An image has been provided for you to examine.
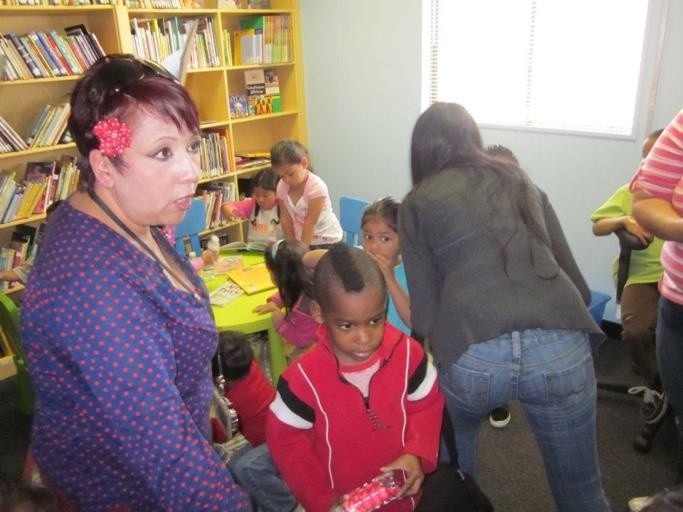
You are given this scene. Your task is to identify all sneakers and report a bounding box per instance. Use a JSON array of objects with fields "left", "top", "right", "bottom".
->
[
  {"left": 488, "top": 406, "right": 512, "bottom": 429},
  {"left": 627, "top": 385, "right": 669, "bottom": 425}
]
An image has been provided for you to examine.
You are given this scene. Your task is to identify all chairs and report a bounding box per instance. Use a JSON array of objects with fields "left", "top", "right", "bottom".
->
[
  {"left": 0, "top": 293, "right": 35, "bottom": 417},
  {"left": 173, "top": 199, "right": 206, "bottom": 259},
  {"left": 596, "top": 226, "right": 668, "bottom": 451},
  {"left": 338, "top": 197, "right": 369, "bottom": 246}
]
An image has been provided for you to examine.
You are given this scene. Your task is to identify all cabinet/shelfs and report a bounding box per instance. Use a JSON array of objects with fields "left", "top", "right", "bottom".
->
[
  {"left": 126, "top": 1, "right": 307, "bottom": 242},
  {"left": 0, "top": 3, "right": 125, "bottom": 384}
]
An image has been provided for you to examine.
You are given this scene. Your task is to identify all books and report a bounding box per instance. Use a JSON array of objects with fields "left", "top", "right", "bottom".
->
[
  {"left": 199, "top": 130, "right": 230, "bottom": 180},
  {"left": 195, "top": 180, "right": 236, "bottom": 229},
  {"left": 129, "top": 17, "right": 222, "bottom": 68},
  {"left": 0, "top": 101, "right": 71, "bottom": 154},
  {"left": 226, "top": 263, "right": 278, "bottom": 295},
  {"left": 220, "top": 240, "right": 269, "bottom": 252},
  {"left": 222, "top": 13, "right": 292, "bottom": 66},
  {"left": 0, "top": 22, "right": 106, "bottom": 81},
  {"left": 1, "top": 225, "right": 39, "bottom": 288},
  {"left": 0, "top": 155, "right": 81, "bottom": 224}
]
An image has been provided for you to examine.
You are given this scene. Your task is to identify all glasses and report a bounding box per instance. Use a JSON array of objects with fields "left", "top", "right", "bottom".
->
[{"left": 86, "top": 56, "right": 181, "bottom": 148}]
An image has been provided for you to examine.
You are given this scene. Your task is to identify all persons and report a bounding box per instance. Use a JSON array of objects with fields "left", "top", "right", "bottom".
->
[
  {"left": 220, "top": 167, "right": 284, "bottom": 241},
  {"left": 253, "top": 238, "right": 319, "bottom": 347},
  {"left": 270, "top": 139, "right": 344, "bottom": 250},
  {"left": 590, "top": 129, "right": 665, "bottom": 390},
  {"left": 265, "top": 242, "right": 444, "bottom": 512},
  {"left": 396, "top": 101, "right": 614, "bottom": 512},
  {"left": 21, "top": 54, "right": 251, "bottom": 512},
  {"left": 0, "top": 199, "right": 64, "bottom": 286},
  {"left": 211, "top": 331, "right": 297, "bottom": 512},
  {"left": 353, "top": 198, "right": 413, "bottom": 339},
  {"left": 483, "top": 145, "right": 590, "bottom": 430},
  {"left": 628, "top": 109, "right": 683, "bottom": 512},
  {"left": 191, "top": 248, "right": 218, "bottom": 273}
]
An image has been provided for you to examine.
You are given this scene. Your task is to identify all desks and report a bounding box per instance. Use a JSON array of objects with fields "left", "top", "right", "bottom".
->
[{"left": 183, "top": 249, "right": 289, "bottom": 389}]
